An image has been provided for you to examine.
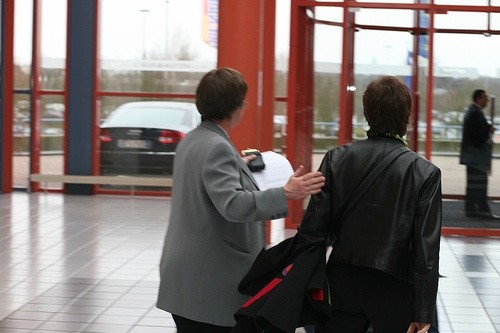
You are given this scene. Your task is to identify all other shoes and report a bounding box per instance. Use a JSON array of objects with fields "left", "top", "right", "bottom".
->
[{"left": 465, "top": 211, "right": 500, "bottom": 220}]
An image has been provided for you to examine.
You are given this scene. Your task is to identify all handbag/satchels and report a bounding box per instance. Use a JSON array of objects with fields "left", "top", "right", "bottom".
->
[{"left": 237, "top": 235, "right": 303, "bottom": 295}]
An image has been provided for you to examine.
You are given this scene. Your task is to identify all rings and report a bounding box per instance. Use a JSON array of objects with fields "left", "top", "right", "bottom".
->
[{"left": 307, "top": 187, "right": 309, "bottom": 191}]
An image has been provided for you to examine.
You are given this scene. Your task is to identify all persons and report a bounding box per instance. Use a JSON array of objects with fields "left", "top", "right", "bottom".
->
[
  {"left": 459, "top": 89, "right": 500, "bottom": 220},
  {"left": 297, "top": 75, "right": 442, "bottom": 333},
  {"left": 155, "top": 68, "right": 326, "bottom": 333}
]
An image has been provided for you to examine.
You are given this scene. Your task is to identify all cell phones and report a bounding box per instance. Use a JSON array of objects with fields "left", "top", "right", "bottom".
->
[{"left": 245, "top": 151, "right": 265, "bottom": 171}]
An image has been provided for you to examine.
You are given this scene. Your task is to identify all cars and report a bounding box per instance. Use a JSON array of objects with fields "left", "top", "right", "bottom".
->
[{"left": 100, "top": 101, "right": 202, "bottom": 178}]
